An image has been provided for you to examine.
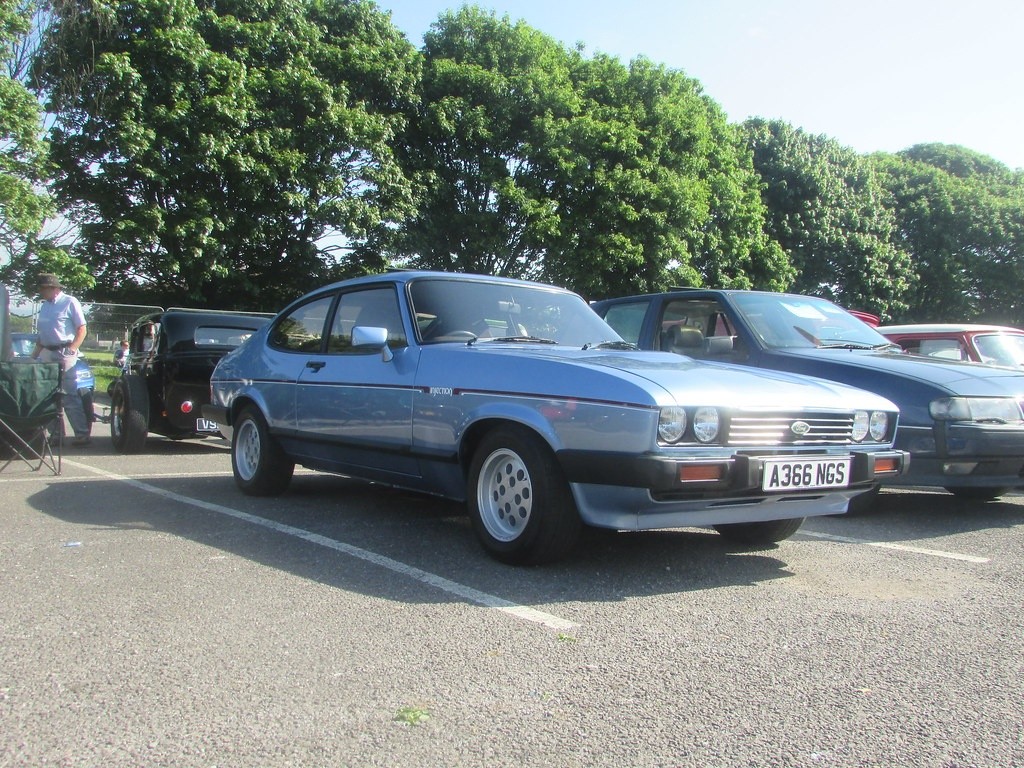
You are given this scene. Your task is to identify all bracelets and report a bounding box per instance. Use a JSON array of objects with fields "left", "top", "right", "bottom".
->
[{"left": 66, "top": 346, "right": 75, "bottom": 355}]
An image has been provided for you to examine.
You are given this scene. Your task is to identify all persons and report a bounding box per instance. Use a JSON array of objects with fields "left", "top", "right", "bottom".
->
[
  {"left": 113, "top": 340, "right": 129, "bottom": 367},
  {"left": 28, "top": 274, "right": 91, "bottom": 446},
  {"left": 143, "top": 310, "right": 155, "bottom": 352}
]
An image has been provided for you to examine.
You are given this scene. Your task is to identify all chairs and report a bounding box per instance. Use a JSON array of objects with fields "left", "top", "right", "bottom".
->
[
  {"left": 354, "top": 298, "right": 408, "bottom": 349},
  {"left": 197, "top": 338, "right": 212, "bottom": 344},
  {"left": 661, "top": 325, "right": 705, "bottom": 361},
  {"left": 1, "top": 359, "right": 65, "bottom": 475},
  {"left": 422, "top": 303, "right": 493, "bottom": 344},
  {"left": 226, "top": 336, "right": 243, "bottom": 345}
]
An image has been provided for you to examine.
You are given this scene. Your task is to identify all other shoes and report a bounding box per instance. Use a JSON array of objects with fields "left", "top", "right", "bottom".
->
[
  {"left": 45, "top": 437, "right": 65, "bottom": 446},
  {"left": 72, "top": 437, "right": 91, "bottom": 446}
]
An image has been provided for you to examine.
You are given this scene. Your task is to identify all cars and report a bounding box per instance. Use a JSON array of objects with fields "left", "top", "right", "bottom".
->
[
  {"left": 588, "top": 290, "right": 1024, "bottom": 520},
  {"left": 196, "top": 269, "right": 914, "bottom": 570},
  {"left": 874, "top": 323, "right": 1024, "bottom": 370},
  {"left": 105, "top": 309, "right": 274, "bottom": 455},
  {"left": 663, "top": 305, "right": 881, "bottom": 337},
  {"left": 12, "top": 332, "right": 96, "bottom": 396}
]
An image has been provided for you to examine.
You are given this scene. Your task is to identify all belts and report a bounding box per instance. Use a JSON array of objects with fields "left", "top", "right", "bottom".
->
[{"left": 42, "top": 342, "right": 72, "bottom": 351}]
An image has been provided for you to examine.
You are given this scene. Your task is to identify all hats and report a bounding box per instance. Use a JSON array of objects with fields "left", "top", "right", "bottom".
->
[{"left": 34, "top": 274, "right": 66, "bottom": 289}]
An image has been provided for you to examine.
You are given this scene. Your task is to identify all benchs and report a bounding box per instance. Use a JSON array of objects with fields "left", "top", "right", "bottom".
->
[{"left": 704, "top": 335, "right": 749, "bottom": 363}]
